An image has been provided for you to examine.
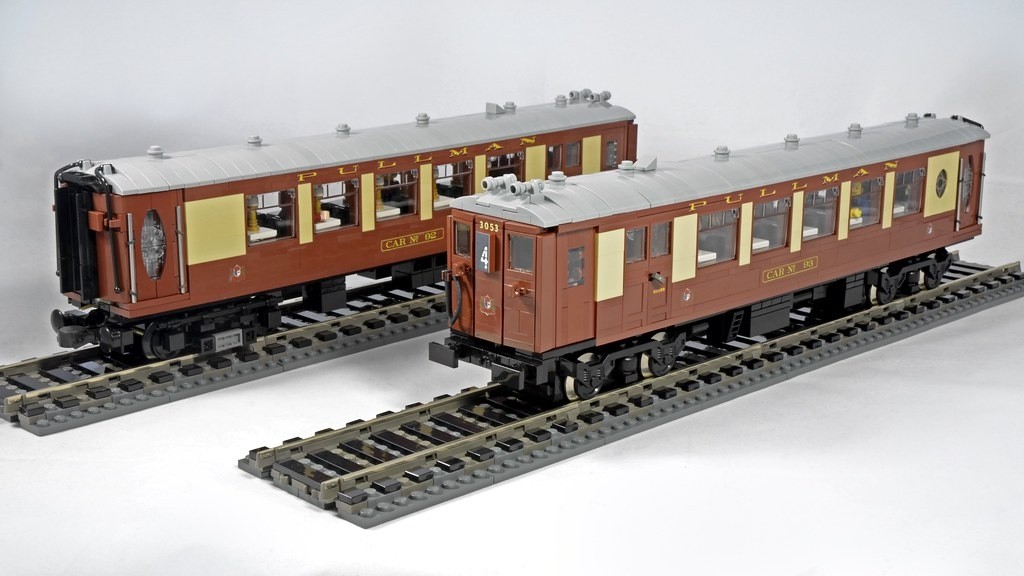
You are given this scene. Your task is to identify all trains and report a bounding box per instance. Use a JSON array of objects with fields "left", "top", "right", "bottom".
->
[
  {"left": 49, "top": 88, "right": 640, "bottom": 364},
  {"left": 428, "top": 112, "right": 991, "bottom": 405}
]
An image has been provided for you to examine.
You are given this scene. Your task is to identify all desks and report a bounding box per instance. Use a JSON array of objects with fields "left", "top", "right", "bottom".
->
[
  {"left": 313, "top": 216, "right": 342, "bottom": 231},
  {"left": 699, "top": 248, "right": 718, "bottom": 262},
  {"left": 247, "top": 225, "right": 277, "bottom": 241},
  {"left": 434, "top": 195, "right": 455, "bottom": 209},
  {"left": 375, "top": 205, "right": 401, "bottom": 218},
  {"left": 751, "top": 237, "right": 771, "bottom": 249},
  {"left": 892, "top": 204, "right": 906, "bottom": 216},
  {"left": 802, "top": 225, "right": 818, "bottom": 238},
  {"left": 851, "top": 216, "right": 863, "bottom": 226}
]
